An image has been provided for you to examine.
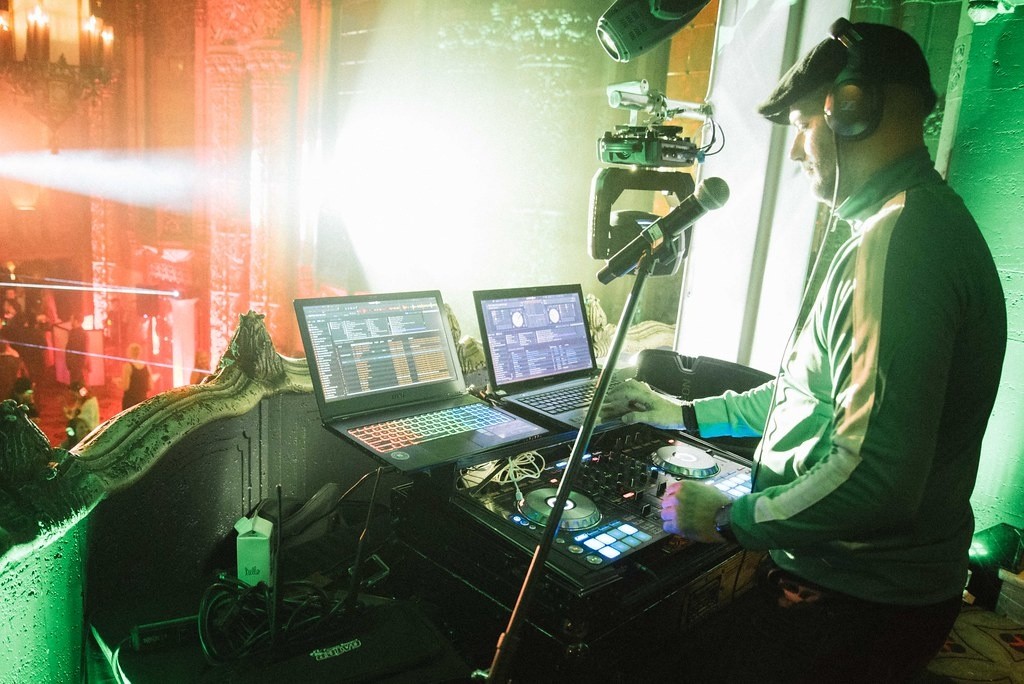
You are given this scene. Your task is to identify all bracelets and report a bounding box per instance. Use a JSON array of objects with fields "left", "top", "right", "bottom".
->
[{"left": 681, "top": 403, "right": 699, "bottom": 433}]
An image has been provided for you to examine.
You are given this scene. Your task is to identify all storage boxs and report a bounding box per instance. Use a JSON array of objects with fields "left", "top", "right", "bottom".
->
[{"left": 238, "top": 508, "right": 276, "bottom": 587}]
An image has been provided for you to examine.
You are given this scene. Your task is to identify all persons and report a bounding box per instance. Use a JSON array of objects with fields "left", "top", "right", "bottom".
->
[
  {"left": 0, "top": 286, "right": 50, "bottom": 408},
  {"left": 599, "top": 17, "right": 1009, "bottom": 684},
  {"left": 111, "top": 341, "right": 153, "bottom": 412},
  {"left": 64, "top": 379, "right": 100, "bottom": 430},
  {"left": 6, "top": 376, "right": 41, "bottom": 424},
  {"left": 64, "top": 310, "right": 94, "bottom": 396},
  {"left": 191, "top": 347, "right": 214, "bottom": 385},
  {"left": 58, "top": 418, "right": 91, "bottom": 451}
]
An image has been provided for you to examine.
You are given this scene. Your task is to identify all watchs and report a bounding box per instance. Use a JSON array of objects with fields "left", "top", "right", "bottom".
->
[{"left": 712, "top": 502, "right": 739, "bottom": 545}]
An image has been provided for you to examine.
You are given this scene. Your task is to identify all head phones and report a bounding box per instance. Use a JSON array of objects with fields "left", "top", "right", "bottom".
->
[{"left": 823, "top": 17, "right": 885, "bottom": 141}]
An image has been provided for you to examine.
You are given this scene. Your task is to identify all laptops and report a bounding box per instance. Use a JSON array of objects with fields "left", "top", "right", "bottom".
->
[
  {"left": 471, "top": 284, "right": 649, "bottom": 431},
  {"left": 293, "top": 290, "right": 549, "bottom": 477}
]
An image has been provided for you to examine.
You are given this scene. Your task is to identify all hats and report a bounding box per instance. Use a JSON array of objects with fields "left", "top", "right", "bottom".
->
[{"left": 759, "top": 22, "right": 932, "bottom": 125}]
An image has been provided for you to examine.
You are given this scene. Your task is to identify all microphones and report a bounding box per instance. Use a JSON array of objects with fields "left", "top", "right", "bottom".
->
[{"left": 596, "top": 177, "right": 730, "bottom": 285}]
